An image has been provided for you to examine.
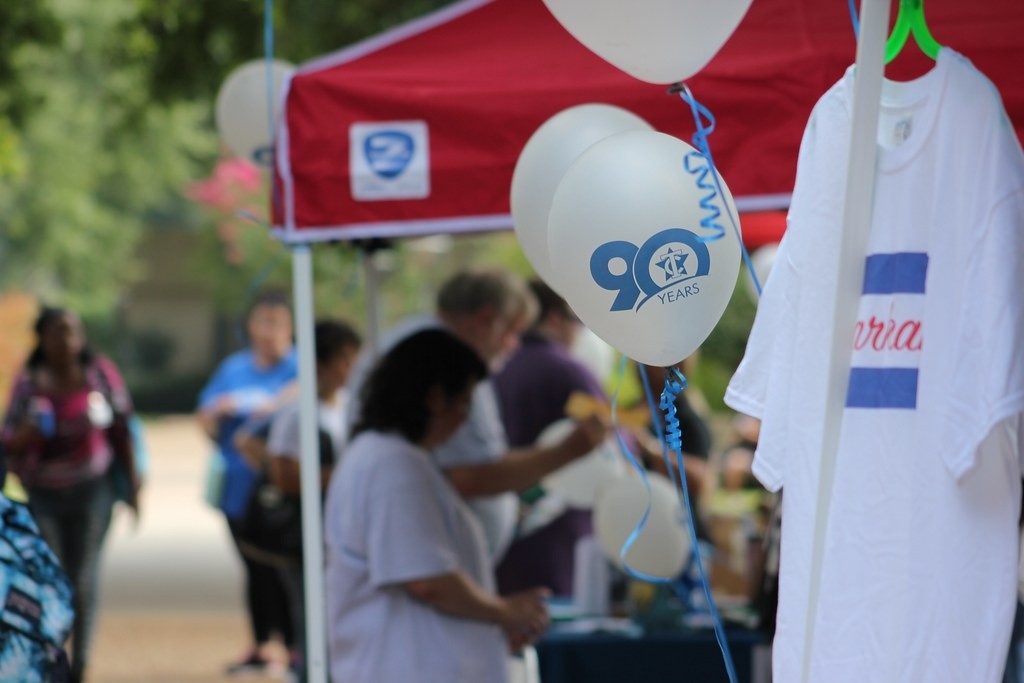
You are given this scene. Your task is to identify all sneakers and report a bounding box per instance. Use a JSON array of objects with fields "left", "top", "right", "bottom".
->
[{"left": 228, "top": 654, "right": 269, "bottom": 678}]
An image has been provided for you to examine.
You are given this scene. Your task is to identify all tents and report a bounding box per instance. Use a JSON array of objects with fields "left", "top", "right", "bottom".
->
[{"left": 269, "top": 1, "right": 1024, "bottom": 244}]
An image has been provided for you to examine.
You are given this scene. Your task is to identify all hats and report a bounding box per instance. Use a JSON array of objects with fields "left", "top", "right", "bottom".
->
[{"left": 530, "top": 279, "right": 584, "bottom": 321}]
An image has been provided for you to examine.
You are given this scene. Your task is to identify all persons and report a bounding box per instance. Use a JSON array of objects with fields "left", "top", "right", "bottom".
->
[
  {"left": 0, "top": 306, "right": 149, "bottom": 681},
  {"left": 194, "top": 270, "right": 778, "bottom": 683},
  {"left": 0, "top": 468, "right": 81, "bottom": 683}
]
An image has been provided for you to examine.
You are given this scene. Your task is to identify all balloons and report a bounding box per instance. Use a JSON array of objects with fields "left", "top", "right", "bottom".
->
[
  {"left": 509, "top": 102, "right": 661, "bottom": 299},
  {"left": 551, "top": 131, "right": 744, "bottom": 367},
  {"left": 543, "top": 0, "right": 751, "bottom": 85},
  {"left": 214, "top": 55, "right": 297, "bottom": 167}
]
